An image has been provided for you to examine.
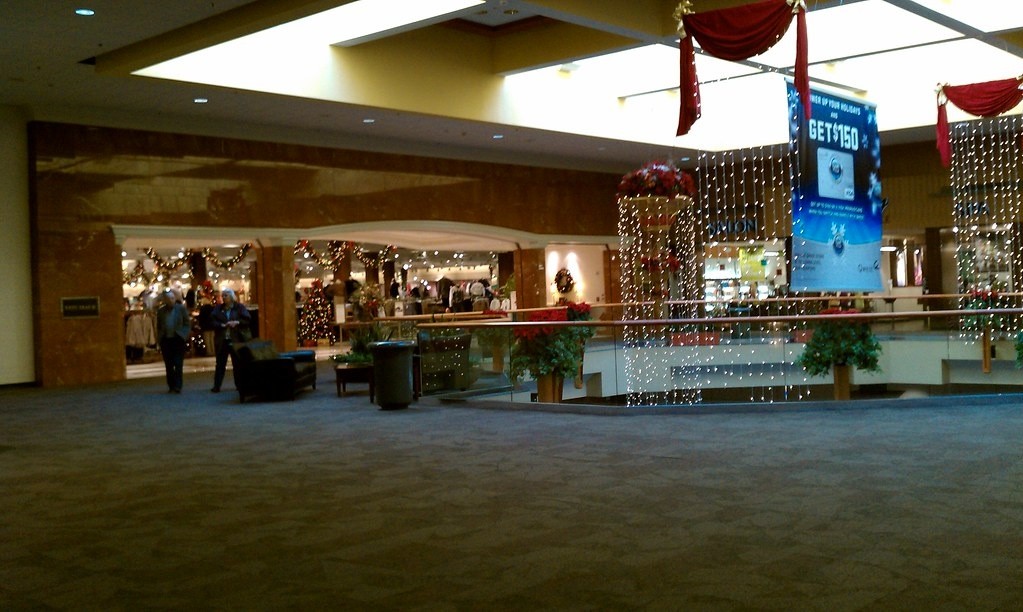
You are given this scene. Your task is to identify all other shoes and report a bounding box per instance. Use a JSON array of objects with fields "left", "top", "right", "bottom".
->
[
  {"left": 168, "top": 387, "right": 182, "bottom": 394},
  {"left": 211, "top": 384, "right": 220, "bottom": 391}
]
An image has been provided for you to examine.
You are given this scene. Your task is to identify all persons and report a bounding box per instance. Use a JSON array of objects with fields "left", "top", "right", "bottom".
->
[
  {"left": 959, "top": 279, "right": 969, "bottom": 307},
  {"left": 211, "top": 288, "right": 250, "bottom": 393},
  {"left": 389, "top": 278, "right": 400, "bottom": 297},
  {"left": 990, "top": 274, "right": 998, "bottom": 292},
  {"left": 156, "top": 292, "right": 189, "bottom": 393}
]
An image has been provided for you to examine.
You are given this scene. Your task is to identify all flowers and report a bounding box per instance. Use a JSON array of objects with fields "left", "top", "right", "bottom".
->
[
  {"left": 615, "top": 160, "right": 696, "bottom": 200},
  {"left": 554, "top": 268, "right": 576, "bottom": 294},
  {"left": 348, "top": 281, "right": 393, "bottom": 317}
]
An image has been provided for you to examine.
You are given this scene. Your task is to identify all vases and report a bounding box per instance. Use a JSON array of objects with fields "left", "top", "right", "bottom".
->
[
  {"left": 621, "top": 196, "right": 692, "bottom": 232},
  {"left": 353, "top": 303, "right": 371, "bottom": 322}
]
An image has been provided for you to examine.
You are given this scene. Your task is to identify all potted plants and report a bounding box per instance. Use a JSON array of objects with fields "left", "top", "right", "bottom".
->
[
  {"left": 664, "top": 325, "right": 720, "bottom": 345},
  {"left": 478, "top": 328, "right": 507, "bottom": 373},
  {"left": 789, "top": 321, "right": 815, "bottom": 342},
  {"left": 507, "top": 316, "right": 598, "bottom": 402}
]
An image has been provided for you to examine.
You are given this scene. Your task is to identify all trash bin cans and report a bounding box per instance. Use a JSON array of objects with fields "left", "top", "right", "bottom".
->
[
  {"left": 728, "top": 308, "right": 753, "bottom": 339},
  {"left": 366, "top": 340, "right": 418, "bottom": 410}
]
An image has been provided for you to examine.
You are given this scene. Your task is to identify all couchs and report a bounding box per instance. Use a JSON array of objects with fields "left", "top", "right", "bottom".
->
[
  {"left": 409, "top": 332, "right": 479, "bottom": 400},
  {"left": 228, "top": 339, "right": 317, "bottom": 405}
]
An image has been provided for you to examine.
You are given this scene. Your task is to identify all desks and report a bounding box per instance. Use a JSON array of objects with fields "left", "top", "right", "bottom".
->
[
  {"left": 340, "top": 321, "right": 401, "bottom": 353},
  {"left": 917, "top": 292, "right": 1023, "bottom": 328}
]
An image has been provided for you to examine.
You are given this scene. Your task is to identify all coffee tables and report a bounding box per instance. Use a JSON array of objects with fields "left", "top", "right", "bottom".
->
[{"left": 335, "top": 362, "right": 372, "bottom": 398}]
